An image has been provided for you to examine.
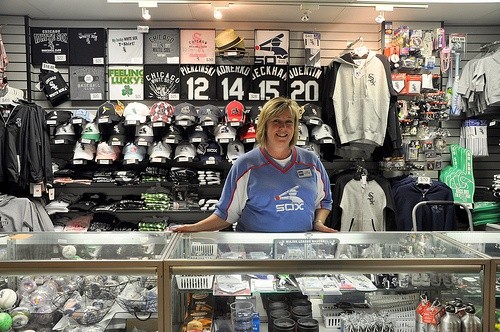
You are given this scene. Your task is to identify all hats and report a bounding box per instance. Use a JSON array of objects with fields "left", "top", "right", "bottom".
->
[
  {"left": 297, "top": 123, "right": 308, "bottom": 140},
  {"left": 298, "top": 104, "right": 323, "bottom": 122},
  {"left": 47, "top": 101, "right": 264, "bottom": 165},
  {"left": 311, "top": 124, "right": 334, "bottom": 141}
]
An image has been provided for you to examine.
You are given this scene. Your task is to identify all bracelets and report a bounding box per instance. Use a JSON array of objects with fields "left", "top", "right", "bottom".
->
[{"left": 314, "top": 220, "right": 321, "bottom": 224}]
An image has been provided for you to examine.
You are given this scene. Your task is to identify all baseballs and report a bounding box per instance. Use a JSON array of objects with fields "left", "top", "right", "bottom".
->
[
  {"left": 0, "top": 288, "right": 17, "bottom": 310},
  {"left": 0, "top": 312, "right": 12, "bottom": 332},
  {"left": 61, "top": 245, "right": 77, "bottom": 259},
  {"left": 9, "top": 307, "right": 31, "bottom": 328}
]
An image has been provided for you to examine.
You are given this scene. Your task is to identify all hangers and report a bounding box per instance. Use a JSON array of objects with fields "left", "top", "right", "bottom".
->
[
  {"left": 408, "top": 165, "right": 433, "bottom": 186},
  {"left": 350, "top": 37, "right": 370, "bottom": 58},
  {"left": 0, "top": 85, "right": 24, "bottom": 107}
]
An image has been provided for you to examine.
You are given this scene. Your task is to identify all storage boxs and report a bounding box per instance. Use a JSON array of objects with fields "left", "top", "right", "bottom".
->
[{"left": 323, "top": 289, "right": 366, "bottom": 305}]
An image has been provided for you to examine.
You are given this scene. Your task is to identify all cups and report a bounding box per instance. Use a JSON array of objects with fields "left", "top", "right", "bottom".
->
[
  {"left": 371, "top": 272, "right": 454, "bottom": 289},
  {"left": 230, "top": 301, "right": 254, "bottom": 332}
]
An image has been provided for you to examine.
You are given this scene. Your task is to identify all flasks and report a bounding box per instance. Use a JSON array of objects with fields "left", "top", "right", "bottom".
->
[{"left": 415, "top": 295, "right": 481, "bottom": 332}]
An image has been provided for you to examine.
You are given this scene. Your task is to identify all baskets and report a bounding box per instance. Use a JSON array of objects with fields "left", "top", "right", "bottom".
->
[
  {"left": 190, "top": 244, "right": 218, "bottom": 260},
  {"left": 319, "top": 303, "right": 372, "bottom": 320},
  {"left": 323, "top": 316, "right": 350, "bottom": 328},
  {"left": 367, "top": 293, "right": 420, "bottom": 332},
  {"left": 175, "top": 275, "right": 214, "bottom": 290}
]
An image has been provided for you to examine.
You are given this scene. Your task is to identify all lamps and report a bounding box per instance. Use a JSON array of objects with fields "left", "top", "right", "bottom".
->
[
  {"left": 298, "top": 7, "right": 312, "bottom": 22},
  {"left": 142, "top": 7, "right": 151, "bottom": 20}
]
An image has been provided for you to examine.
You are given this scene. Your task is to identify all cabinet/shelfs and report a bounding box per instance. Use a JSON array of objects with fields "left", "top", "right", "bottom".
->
[{"left": 54, "top": 180, "right": 229, "bottom": 214}]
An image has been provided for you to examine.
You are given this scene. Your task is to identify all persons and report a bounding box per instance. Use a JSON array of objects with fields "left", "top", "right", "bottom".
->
[{"left": 171, "top": 97, "right": 337, "bottom": 233}]
